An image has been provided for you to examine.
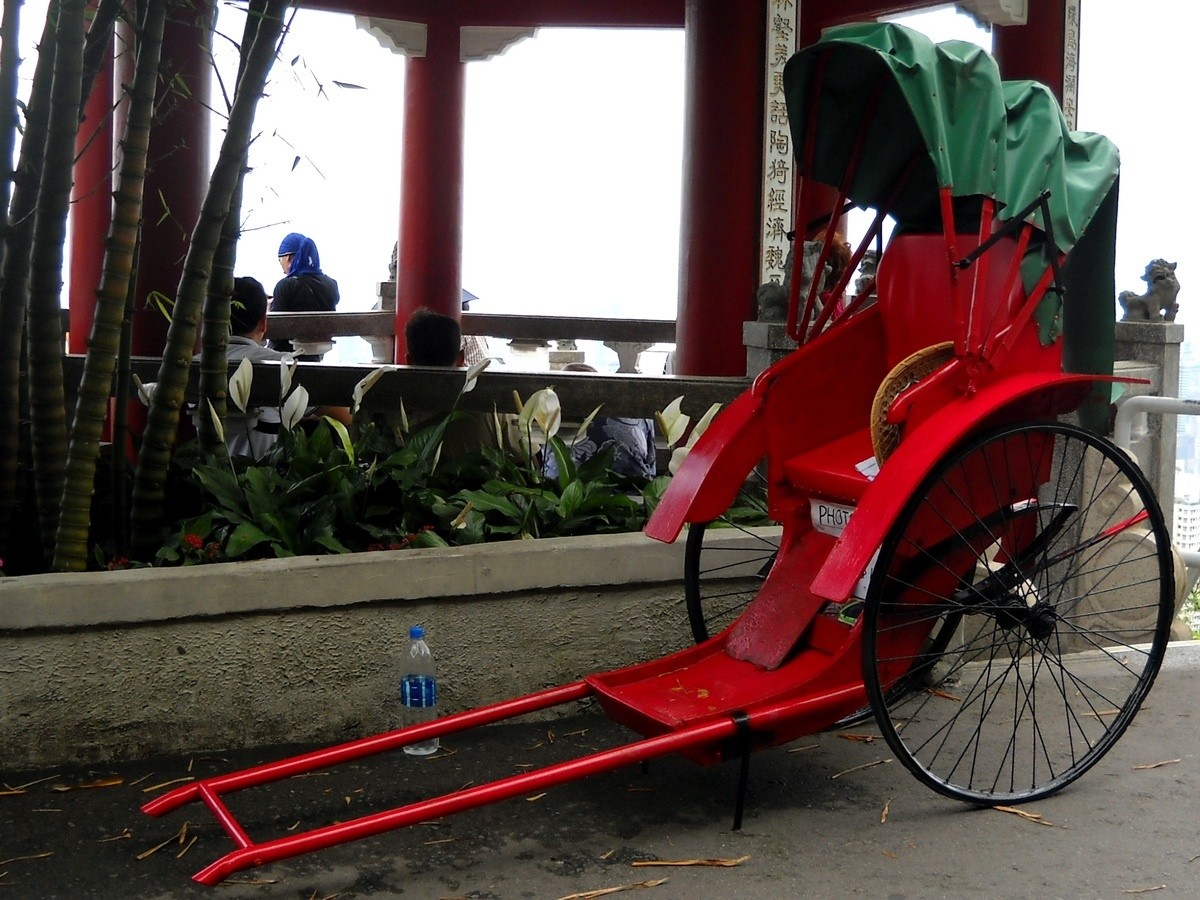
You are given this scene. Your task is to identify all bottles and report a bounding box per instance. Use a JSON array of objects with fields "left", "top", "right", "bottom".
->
[{"left": 400, "top": 626, "right": 440, "bottom": 755}]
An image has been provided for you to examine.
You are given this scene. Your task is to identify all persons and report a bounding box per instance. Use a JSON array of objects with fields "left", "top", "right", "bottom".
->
[
  {"left": 265, "top": 232, "right": 340, "bottom": 362},
  {"left": 814, "top": 229, "right": 850, "bottom": 321},
  {"left": 188, "top": 277, "right": 658, "bottom": 481}
]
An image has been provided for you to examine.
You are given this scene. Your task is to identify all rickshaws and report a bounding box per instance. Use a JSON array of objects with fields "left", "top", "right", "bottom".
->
[{"left": 140, "top": 21, "right": 1177, "bottom": 889}]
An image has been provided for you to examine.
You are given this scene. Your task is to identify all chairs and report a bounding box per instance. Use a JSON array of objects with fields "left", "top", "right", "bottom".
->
[{"left": 753, "top": 234, "right": 1021, "bottom": 501}]
